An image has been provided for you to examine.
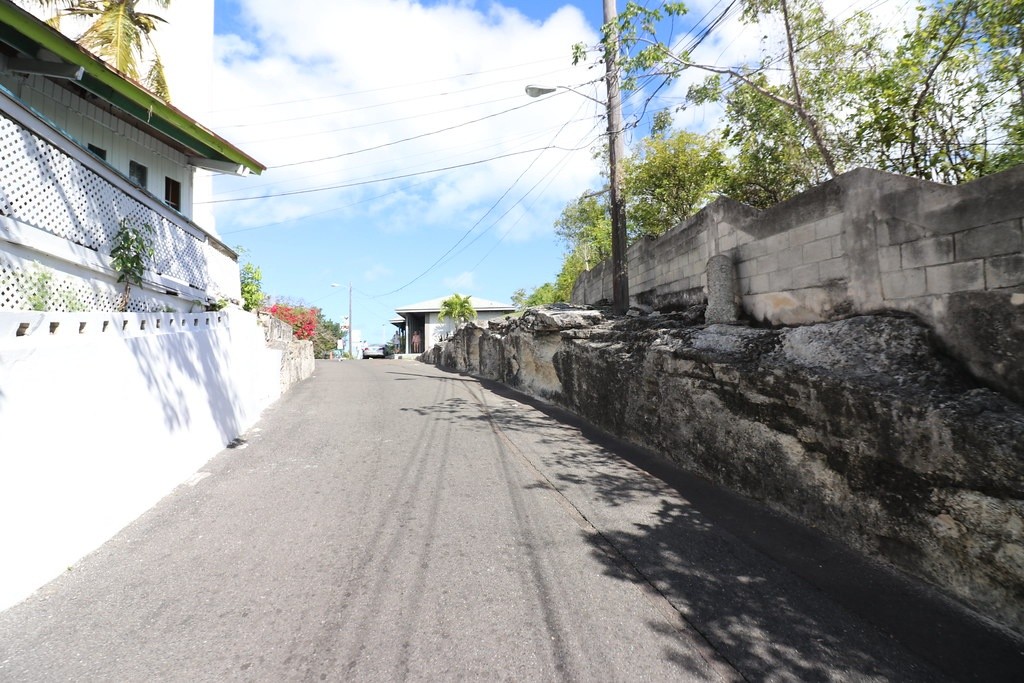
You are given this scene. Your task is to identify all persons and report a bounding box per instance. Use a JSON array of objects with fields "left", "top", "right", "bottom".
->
[
  {"left": 356, "top": 341, "right": 368, "bottom": 359},
  {"left": 337, "top": 337, "right": 344, "bottom": 362},
  {"left": 394, "top": 330, "right": 400, "bottom": 354},
  {"left": 411, "top": 330, "right": 420, "bottom": 353}
]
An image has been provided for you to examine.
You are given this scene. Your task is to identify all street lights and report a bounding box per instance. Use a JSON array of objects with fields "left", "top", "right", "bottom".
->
[
  {"left": 330, "top": 281, "right": 354, "bottom": 358},
  {"left": 524, "top": 83, "right": 630, "bottom": 315}
]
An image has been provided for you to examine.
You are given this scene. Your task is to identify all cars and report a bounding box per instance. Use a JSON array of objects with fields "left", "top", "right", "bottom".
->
[{"left": 362, "top": 344, "right": 385, "bottom": 360}]
